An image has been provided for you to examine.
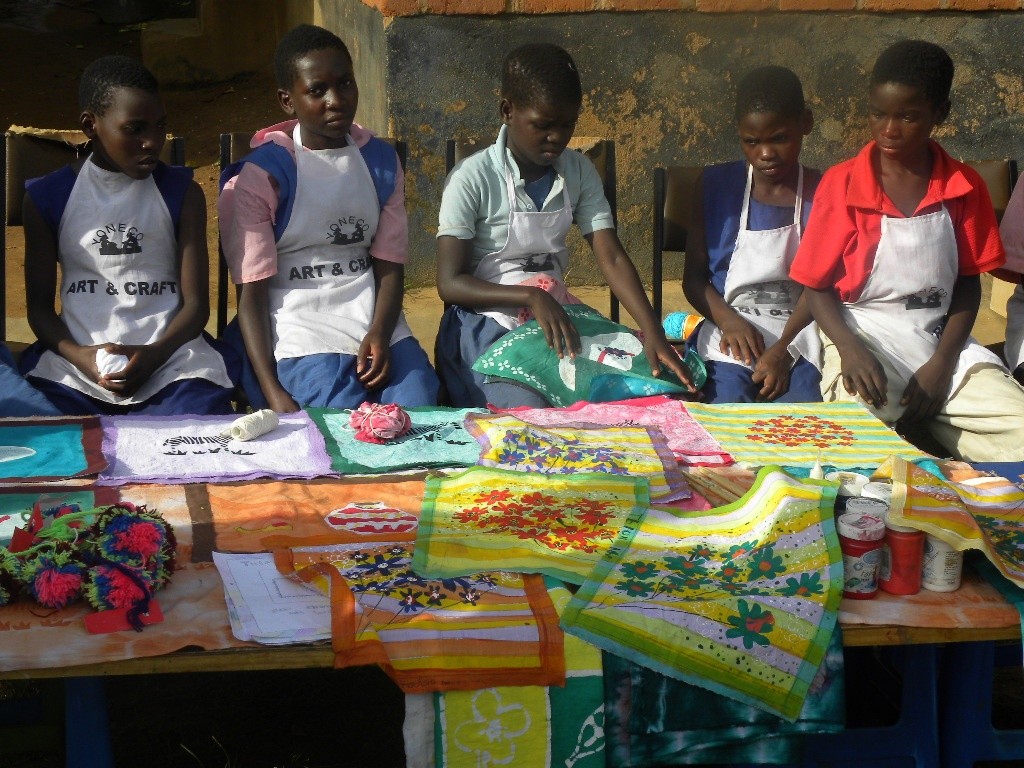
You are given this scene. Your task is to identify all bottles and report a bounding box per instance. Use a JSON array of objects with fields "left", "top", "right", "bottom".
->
[{"left": 810, "top": 460, "right": 965, "bottom": 600}]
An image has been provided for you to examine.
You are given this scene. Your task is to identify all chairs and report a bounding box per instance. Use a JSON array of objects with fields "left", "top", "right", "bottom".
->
[
  {"left": 5, "top": 123, "right": 188, "bottom": 379},
  {"left": 651, "top": 162, "right": 706, "bottom": 375},
  {"left": 443, "top": 137, "right": 621, "bottom": 328},
  {"left": 956, "top": 155, "right": 1020, "bottom": 372},
  {"left": 216, "top": 131, "right": 406, "bottom": 419}
]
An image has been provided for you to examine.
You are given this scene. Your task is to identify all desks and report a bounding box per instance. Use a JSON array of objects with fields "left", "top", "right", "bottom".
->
[{"left": 0, "top": 393, "right": 1022, "bottom": 768}]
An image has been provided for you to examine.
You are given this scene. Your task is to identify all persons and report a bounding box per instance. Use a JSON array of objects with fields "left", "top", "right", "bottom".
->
[
  {"left": 216, "top": 22, "right": 443, "bottom": 414},
  {"left": 680, "top": 64, "right": 828, "bottom": 404},
  {"left": 433, "top": 40, "right": 706, "bottom": 409},
  {"left": 22, "top": 53, "right": 245, "bottom": 418},
  {"left": 984, "top": 165, "right": 1024, "bottom": 386},
  {"left": 786, "top": 38, "right": 1024, "bottom": 462}
]
